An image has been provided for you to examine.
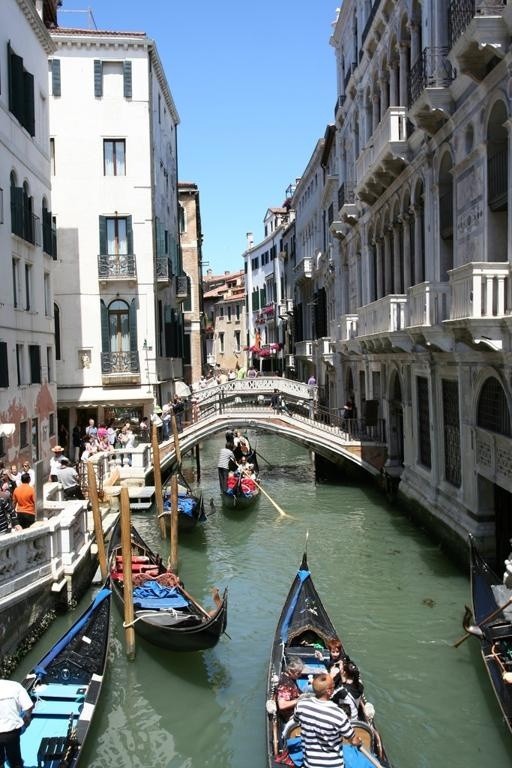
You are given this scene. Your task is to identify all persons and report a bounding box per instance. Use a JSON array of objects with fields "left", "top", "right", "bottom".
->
[
  {"left": 1, "top": 679, "right": 34, "bottom": 767},
  {"left": 273, "top": 637, "right": 370, "bottom": 768},
  {"left": 269, "top": 388, "right": 293, "bottom": 418},
  {"left": 341, "top": 396, "right": 354, "bottom": 433},
  {"left": 308, "top": 375, "right": 316, "bottom": 385}
]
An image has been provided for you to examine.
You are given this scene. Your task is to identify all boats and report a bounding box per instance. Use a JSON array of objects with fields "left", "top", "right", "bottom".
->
[
  {"left": 462, "top": 531, "right": 511, "bottom": 734},
  {"left": 221, "top": 438, "right": 260, "bottom": 510},
  {"left": 157, "top": 462, "right": 202, "bottom": 531},
  {"left": 265, "top": 530, "right": 390, "bottom": 768},
  {"left": 0, "top": 575, "right": 113, "bottom": 768},
  {"left": 106, "top": 513, "right": 227, "bottom": 652}
]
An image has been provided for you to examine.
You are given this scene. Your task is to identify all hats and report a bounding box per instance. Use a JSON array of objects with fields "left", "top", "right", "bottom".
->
[{"left": 51, "top": 445, "right": 65, "bottom": 452}]
